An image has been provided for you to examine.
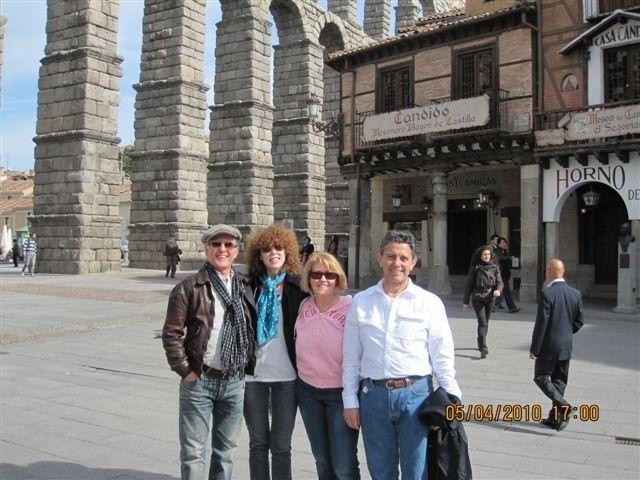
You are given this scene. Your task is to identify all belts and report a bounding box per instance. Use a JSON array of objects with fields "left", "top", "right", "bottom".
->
[
  {"left": 372, "top": 375, "right": 420, "bottom": 390},
  {"left": 202, "top": 364, "right": 230, "bottom": 380}
]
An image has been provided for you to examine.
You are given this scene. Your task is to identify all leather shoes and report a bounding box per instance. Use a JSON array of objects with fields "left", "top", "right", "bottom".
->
[
  {"left": 556, "top": 401, "right": 574, "bottom": 432},
  {"left": 540, "top": 417, "right": 557, "bottom": 429}
]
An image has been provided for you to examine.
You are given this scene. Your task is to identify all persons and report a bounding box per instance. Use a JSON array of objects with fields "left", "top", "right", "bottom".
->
[
  {"left": 295, "top": 252, "right": 362, "bottom": 480},
  {"left": 496, "top": 237, "right": 522, "bottom": 313},
  {"left": 328, "top": 235, "right": 339, "bottom": 258},
  {"left": 162, "top": 223, "right": 259, "bottom": 480},
  {"left": 342, "top": 229, "right": 462, "bottom": 480},
  {"left": 300, "top": 237, "right": 314, "bottom": 263},
  {"left": 490, "top": 235, "right": 500, "bottom": 244},
  {"left": 21, "top": 234, "right": 37, "bottom": 277},
  {"left": 243, "top": 225, "right": 312, "bottom": 480},
  {"left": 529, "top": 259, "right": 584, "bottom": 432},
  {"left": 463, "top": 246, "right": 504, "bottom": 358},
  {"left": 12, "top": 239, "right": 18, "bottom": 267},
  {"left": 163, "top": 234, "right": 182, "bottom": 278}
]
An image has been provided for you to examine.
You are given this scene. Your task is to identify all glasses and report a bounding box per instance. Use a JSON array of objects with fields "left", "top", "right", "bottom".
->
[
  {"left": 309, "top": 270, "right": 339, "bottom": 280},
  {"left": 259, "top": 244, "right": 285, "bottom": 254},
  {"left": 206, "top": 240, "right": 238, "bottom": 249}
]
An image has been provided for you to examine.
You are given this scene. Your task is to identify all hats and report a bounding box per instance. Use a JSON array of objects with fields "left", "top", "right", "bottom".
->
[{"left": 200, "top": 222, "right": 243, "bottom": 245}]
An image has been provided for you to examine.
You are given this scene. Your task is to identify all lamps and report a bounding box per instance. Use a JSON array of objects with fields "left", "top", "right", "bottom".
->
[
  {"left": 583, "top": 183, "right": 600, "bottom": 207},
  {"left": 392, "top": 164, "right": 402, "bottom": 211},
  {"left": 488, "top": 192, "right": 499, "bottom": 235},
  {"left": 479, "top": 164, "right": 491, "bottom": 209},
  {"left": 421, "top": 197, "right": 432, "bottom": 252}
]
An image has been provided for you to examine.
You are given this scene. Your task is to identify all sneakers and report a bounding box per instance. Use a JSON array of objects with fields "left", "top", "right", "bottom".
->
[
  {"left": 508, "top": 306, "right": 522, "bottom": 313},
  {"left": 494, "top": 304, "right": 505, "bottom": 309},
  {"left": 481, "top": 346, "right": 489, "bottom": 359}
]
